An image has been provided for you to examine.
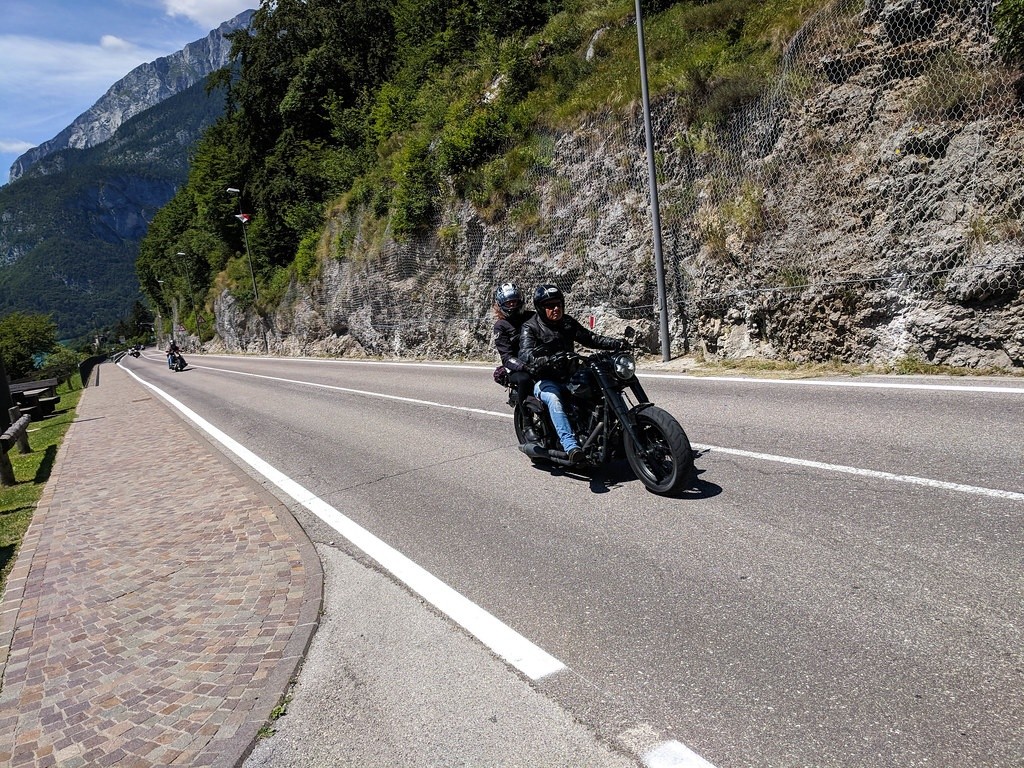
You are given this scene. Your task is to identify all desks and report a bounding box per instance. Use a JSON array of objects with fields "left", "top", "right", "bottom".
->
[{"left": 23, "top": 388, "right": 49, "bottom": 399}]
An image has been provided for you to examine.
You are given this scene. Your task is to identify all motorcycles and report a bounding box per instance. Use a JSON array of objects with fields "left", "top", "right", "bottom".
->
[
  {"left": 494, "top": 325, "right": 695, "bottom": 496},
  {"left": 167, "top": 350, "right": 183, "bottom": 372}
]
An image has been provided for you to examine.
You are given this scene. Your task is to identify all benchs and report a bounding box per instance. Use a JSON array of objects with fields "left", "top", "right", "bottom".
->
[{"left": 38, "top": 397, "right": 61, "bottom": 414}]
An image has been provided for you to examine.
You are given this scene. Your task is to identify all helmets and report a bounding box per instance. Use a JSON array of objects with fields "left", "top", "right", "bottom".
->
[
  {"left": 496, "top": 283, "right": 526, "bottom": 317},
  {"left": 533, "top": 286, "right": 565, "bottom": 326},
  {"left": 169, "top": 340, "right": 175, "bottom": 346}
]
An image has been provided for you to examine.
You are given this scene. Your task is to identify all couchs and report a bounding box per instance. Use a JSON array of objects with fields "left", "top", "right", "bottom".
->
[{"left": 11, "top": 391, "right": 43, "bottom": 422}]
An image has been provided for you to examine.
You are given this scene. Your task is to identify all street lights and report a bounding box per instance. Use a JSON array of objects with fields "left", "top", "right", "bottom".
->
[
  {"left": 225, "top": 188, "right": 271, "bottom": 355},
  {"left": 176, "top": 252, "right": 203, "bottom": 345}
]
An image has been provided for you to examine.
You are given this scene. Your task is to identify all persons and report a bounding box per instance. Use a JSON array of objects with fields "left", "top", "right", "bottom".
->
[
  {"left": 517, "top": 285, "right": 634, "bottom": 471},
  {"left": 491, "top": 283, "right": 540, "bottom": 394},
  {"left": 166, "top": 339, "right": 189, "bottom": 369},
  {"left": 128, "top": 344, "right": 144, "bottom": 358}
]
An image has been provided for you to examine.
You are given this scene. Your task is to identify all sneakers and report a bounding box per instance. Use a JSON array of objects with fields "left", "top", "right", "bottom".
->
[
  {"left": 522, "top": 426, "right": 539, "bottom": 441},
  {"left": 568, "top": 448, "right": 583, "bottom": 462}
]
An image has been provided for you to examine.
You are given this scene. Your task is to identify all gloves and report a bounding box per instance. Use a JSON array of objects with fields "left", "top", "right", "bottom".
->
[
  {"left": 614, "top": 338, "right": 631, "bottom": 352},
  {"left": 533, "top": 355, "right": 552, "bottom": 374}
]
